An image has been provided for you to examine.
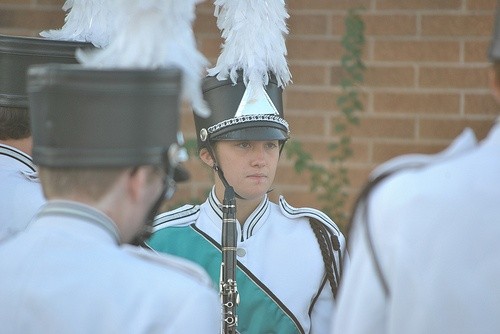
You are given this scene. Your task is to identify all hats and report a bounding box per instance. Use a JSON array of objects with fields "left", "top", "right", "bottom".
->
[
  {"left": 191, "top": 0, "right": 292, "bottom": 143},
  {"left": 23, "top": 0, "right": 186, "bottom": 172},
  {"left": 0, "top": 33, "right": 93, "bottom": 110}
]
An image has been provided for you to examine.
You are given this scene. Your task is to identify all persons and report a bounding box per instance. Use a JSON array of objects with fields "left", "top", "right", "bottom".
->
[
  {"left": 139, "top": 0, "right": 346, "bottom": 334},
  {"left": 0, "top": 0, "right": 500, "bottom": 334}
]
